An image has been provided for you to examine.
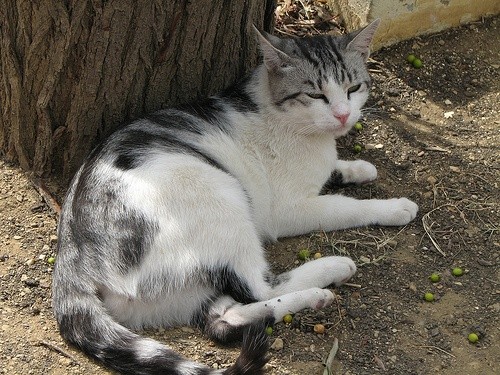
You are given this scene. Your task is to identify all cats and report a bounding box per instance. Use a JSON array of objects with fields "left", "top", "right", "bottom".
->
[{"left": 51, "top": 23, "right": 421, "bottom": 375}]
